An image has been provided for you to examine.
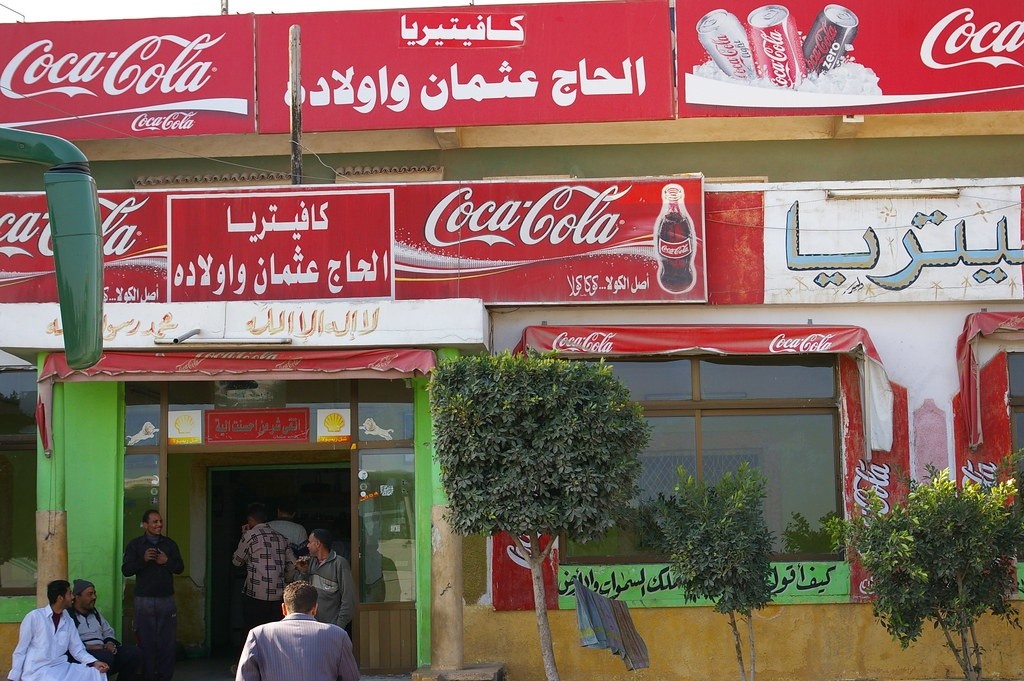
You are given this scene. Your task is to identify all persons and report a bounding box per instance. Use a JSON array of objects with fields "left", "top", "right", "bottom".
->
[
  {"left": 235, "top": 580, "right": 360, "bottom": 681},
  {"left": 66, "top": 578, "right": 144, "bottom": 681},
  {"left": 295, "top": 528, "right": 358, "bottom": 633},
  {"left": 6, "top": 579, "right": 111, "bottom": 681},
  {"left": 122, "top": 509, "right": 185, "bottom": 681},
  {"left": 230, "top": 499, "right": 309, "bottom": 675}
]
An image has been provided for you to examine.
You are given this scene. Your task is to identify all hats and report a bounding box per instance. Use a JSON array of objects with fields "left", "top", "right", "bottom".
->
[{"left": 73, "top": 579, "right": 94, "bottom": 595}]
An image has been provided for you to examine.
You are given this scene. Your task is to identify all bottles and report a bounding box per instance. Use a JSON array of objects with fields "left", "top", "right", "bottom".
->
[{"left": 655, "top": 184, "right": 697, "bottom": 293}]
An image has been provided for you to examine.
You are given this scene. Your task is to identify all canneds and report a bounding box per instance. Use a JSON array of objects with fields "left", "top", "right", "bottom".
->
[
  {"left": 747, "top": 4, "right": 808, "bottom": 88},
  {"left": 696, "top": 9, "right": 756, "bottom": 80},
  {"left": 802, "top": 4, "right": 859, "bottom": 78}
]
